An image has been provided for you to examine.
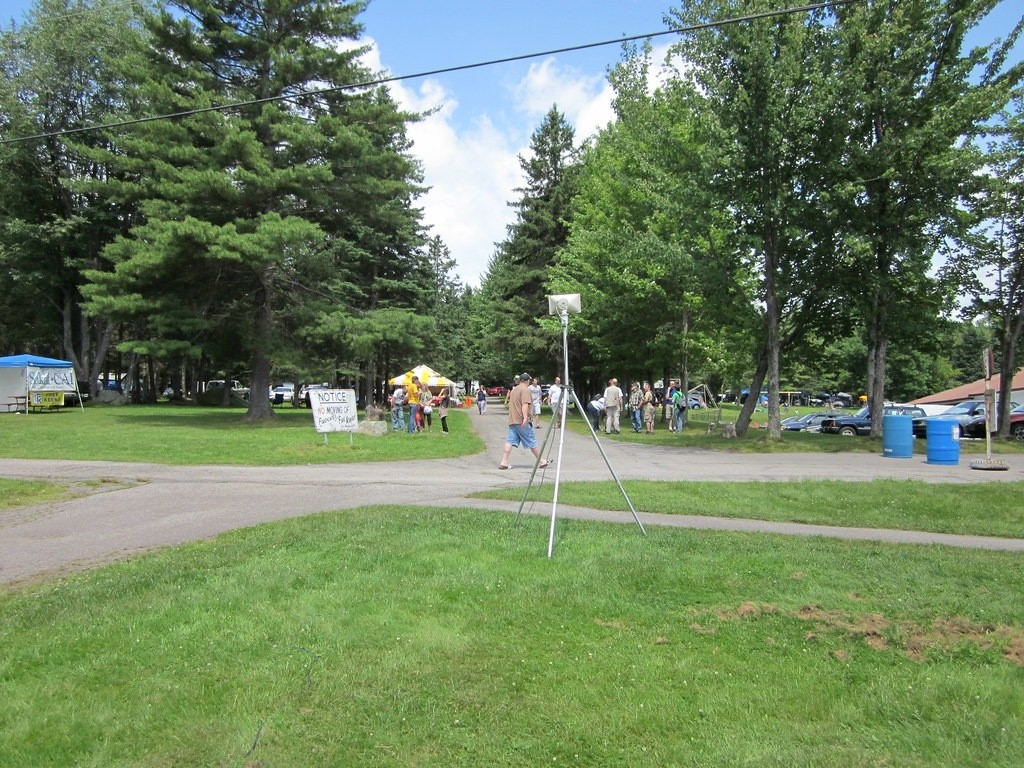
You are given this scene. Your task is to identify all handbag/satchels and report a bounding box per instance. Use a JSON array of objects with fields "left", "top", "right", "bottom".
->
[{"left": 423, "top": 406, "right": 433, "bottom": 414}]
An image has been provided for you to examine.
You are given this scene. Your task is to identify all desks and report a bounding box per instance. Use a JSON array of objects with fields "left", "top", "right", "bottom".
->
[{"left": 8, "top": 396, "right": 37, "bottom": 413}]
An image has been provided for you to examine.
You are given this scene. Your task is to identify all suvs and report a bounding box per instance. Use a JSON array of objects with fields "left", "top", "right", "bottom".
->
[{"left": 206, "top": 380, "right": 250, "bottom": 399}]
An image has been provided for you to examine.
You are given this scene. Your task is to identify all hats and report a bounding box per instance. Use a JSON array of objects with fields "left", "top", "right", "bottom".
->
[
  {"left": 514, "top": 375, "right": 520, "bottom": 380},
  {"left": 519, "top": 373, "right": 532, "bottom": 381}
]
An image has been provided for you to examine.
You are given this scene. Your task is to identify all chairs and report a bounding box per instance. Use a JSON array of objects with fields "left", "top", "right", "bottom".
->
[{"left": 272, "top": 393, "right": 284, "bottom": 408}]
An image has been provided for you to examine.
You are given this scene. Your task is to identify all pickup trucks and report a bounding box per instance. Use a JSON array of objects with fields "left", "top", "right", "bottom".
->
[{"left": 60, "top": 380, "right": 92, "bottom": 406}]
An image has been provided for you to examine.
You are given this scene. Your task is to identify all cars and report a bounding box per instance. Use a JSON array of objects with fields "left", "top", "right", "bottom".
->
[
  {"left": 774, "top": 399, "right": 1024, "bottom": 439},
  {"left": 488, "top": 386, "right": 508, "bottom": 396},
  {"left": 301, "top": 386, "right": 322, "bottom": 398},
  {"left": 95, "top": 372, "right": 130, "bottom": 392},
  {"left": 688, "top": 391, "right": 708, "bottom": 410},
  {"left": 271, "top": 386, "right": 295, "bottom": 402}
]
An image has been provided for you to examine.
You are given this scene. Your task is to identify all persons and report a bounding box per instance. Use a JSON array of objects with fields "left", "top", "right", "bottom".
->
[
  {"left": 475, "top": 384, "right": 487, "bottom": 415},
  {"left": 499, "top": 373, "right": 554, "bottom": 470},
  {"left": 529, "top": 378, "right": 542, "bottom": 428},
  {"left": 588, "top": 378, "right": 687, "bottom": 435},
  {"left": 780, "top": 399, "right": 790, "bottom": 413},
  {"left": 505, "top": 375, "right": 520, "bottom": 406},
  {"left": 548, "top": 377, "right": 565, "bottom": 428},
  {"left": 390, "top": 376, "right": 450, "bottom": 435}
]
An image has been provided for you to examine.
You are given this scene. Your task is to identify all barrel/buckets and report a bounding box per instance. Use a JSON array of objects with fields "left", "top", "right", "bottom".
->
[
  {"left": 882, "top": 415, "right": 913, "bottom": 457},
  {"left": 926, "top": 418, "right": 959, "bottom": 465}
]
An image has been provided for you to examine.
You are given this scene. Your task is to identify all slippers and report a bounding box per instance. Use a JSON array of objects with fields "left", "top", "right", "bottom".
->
[
  {"left": 499, "top": 465, "right": 512, "bottom": 470},
  {"left": 540, "top": 459, "right": 553, "bottom": 468}
]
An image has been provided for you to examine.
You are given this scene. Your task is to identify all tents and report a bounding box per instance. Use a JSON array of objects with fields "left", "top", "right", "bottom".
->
[
  {"left": 0, "top": 354, "right": 85, "bottom": 414},
  {"left": 815, "top": 391, "right": 852, "bottom": 408},
  {"left": 383, "top": 365, "right": 457, "bottom": 406}
]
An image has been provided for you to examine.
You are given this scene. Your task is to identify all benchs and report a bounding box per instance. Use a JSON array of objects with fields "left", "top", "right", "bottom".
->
[{"left": 0, "top": 403, "right": 32, "bottom": 406}]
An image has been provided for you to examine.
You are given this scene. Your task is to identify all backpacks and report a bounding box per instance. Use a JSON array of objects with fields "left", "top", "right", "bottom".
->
[
  {"left": 647, "top": 391, "right": 656, "bottom": 406},
  {"left": 676, "top": 392, "right": 686, "bottom": 411}
]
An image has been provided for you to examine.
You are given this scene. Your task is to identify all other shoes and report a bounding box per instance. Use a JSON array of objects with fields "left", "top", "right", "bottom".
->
[
  {"left": 606, "top": 432, "right": 610, "bottom": 434},
  {"left": 673, "top": 428, "right": 676, "bottom": 432},
  {"left": 534, "top": 425, "right": 541, "bottom": 429},
  {"left": 614, "top": 429, "right": 619, "bottom": 434},
  {"left": 646, "top": 430, "right": 650, "bottom": 433},
  {"left": 441, "top": 430, "right": 448, "bottom": 435},
  {"left": 669, "top": 427, "right": 673, "bottom": 432}
]
